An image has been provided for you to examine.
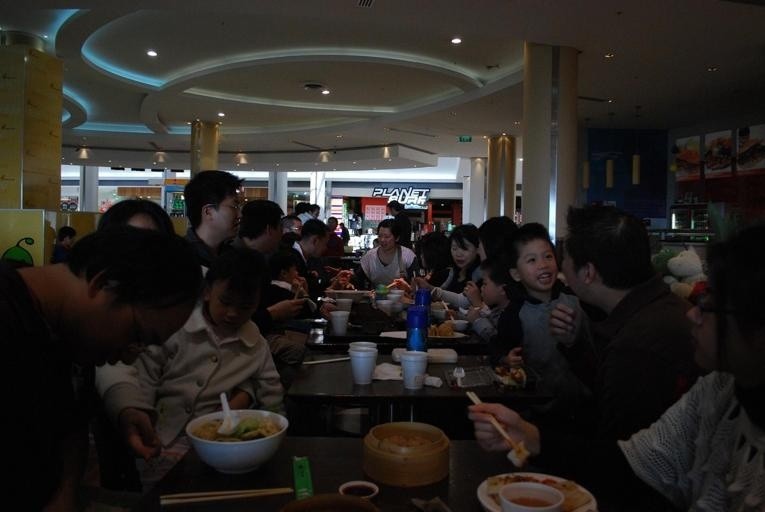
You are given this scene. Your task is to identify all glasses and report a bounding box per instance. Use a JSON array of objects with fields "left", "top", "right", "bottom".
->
[
  {"left": 126, "top": 297, "right": 151, "bottom": 357},
  {"left": 287, "top": 226, "right": 302, "bottom": 232},
  {"left": 696, "top": 292, "right": 738, "bottom": 318},
  {"left": 206, "top": 201, "right": 242, "bottom": 210}
]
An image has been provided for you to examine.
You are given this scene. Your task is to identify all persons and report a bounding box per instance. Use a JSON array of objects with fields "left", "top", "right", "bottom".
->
[
  {"left": 2, "top": 170, "right": 350, "bottom": 511},
  {"left": 354, "top": 200, "right": 517, "bottom": 308},
  {"left": 462, "top": 206, "right": 765, "bottom": 510}
]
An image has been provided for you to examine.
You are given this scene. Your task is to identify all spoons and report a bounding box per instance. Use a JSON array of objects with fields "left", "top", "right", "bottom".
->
[{"left": 215, "top": 391, "right": 236, "bottom": 435}]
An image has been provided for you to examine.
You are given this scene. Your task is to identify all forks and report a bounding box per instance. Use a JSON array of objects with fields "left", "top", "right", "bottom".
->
[{"left": 452, "top": 366, "right": 468, "bottom": 387}]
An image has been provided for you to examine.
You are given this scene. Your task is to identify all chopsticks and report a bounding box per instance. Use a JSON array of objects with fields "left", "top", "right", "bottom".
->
[
  {"left": 464, "top": 389, "right": 525, "bottom": 457},
  {"left": 440, "top": 298, "right": 456, "bottom": 322},
  {"left": 159, "top": 486, "right": 295, "bottom": 504}
]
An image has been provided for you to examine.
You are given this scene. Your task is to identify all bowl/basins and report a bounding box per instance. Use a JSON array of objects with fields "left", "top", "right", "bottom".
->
[
  {"left": 184, "top": 410, "right": 289, "bottom": 475},
  {"left": 445, "top": 318, "right": 469, "bottom": 329},
  {"left": 431, "top": 309, "right": 451, "bottom": 320},
  {"left": 498, "top": 479, "right": 566, "bottom": 511},
  {"left": 325, "top": 289, "right": 365, "bottom": 303}
]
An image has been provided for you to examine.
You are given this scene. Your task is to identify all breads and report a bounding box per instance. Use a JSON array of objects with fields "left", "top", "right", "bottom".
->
[{"left": 428, "top": 323, "right": 455, "bottom": 337}]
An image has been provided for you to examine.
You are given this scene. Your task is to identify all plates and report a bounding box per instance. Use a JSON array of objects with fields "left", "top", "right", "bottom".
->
[
  {"left": 477, "top": 472, "right": 598, "bottom": 511},
  {"left": 338, "top": 479, "right": 379, "bottom": 499},
  {"left": 425, "top": 327, "right": 465, "bottom": 339}
]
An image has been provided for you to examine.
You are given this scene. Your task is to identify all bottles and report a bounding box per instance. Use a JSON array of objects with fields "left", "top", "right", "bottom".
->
[
  {"left": 170, "top": 193, "right": 185, "bottom": 225},
  {"left": 405, "top": 304, "right": 429, "bottom": 350},
  {"left": 415, "top": 288, "right": 432, "bottom": 310}
]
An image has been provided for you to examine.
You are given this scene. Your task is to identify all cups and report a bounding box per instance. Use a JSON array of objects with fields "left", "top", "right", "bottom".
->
[
  {"left": 333, "top": 298, "right": 352, "bottom": 311},
  {"left": 399, "top": 349, "right": 429, "bottom": 389},
  {"left": 375, "top": 281, "right": 404, "bottom": 316},
  {"left": 329, "top": 310, "right": 351, "bottom": 337},
  {"left": 349, "top": 340, "right": 378, "bottom": 384}
]
